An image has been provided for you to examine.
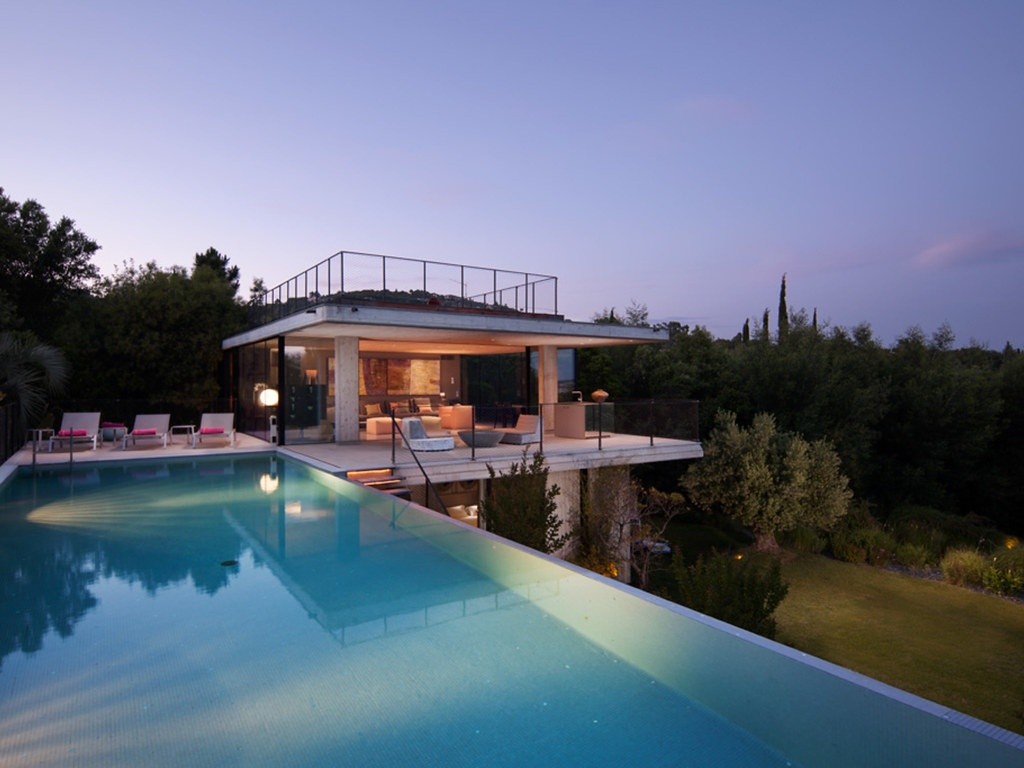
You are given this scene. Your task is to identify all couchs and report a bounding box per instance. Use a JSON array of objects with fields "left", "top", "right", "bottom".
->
[
  {"left": 326, "top": 394, "right": 443, "bottom": 424},
  {"left": 438, "top": 405, "right": 472, "bottom": 429},
  {"left": 491, "top": 415, "right": 545, "bottom": 444}
]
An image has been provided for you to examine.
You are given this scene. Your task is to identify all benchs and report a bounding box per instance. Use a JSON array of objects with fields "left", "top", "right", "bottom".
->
[
  {"left": 366, "top": 417, "right": 401, "bottom": 434},
  {"left": 421, "top": 416, "right": 441, "bottom": 430}
]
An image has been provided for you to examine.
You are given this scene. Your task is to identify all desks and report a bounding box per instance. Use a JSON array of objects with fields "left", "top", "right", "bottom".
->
[
  {"left": 169, "top": 425, "right": 194, "bottom": 445},
  {"left": 459, "top": 430, "right": 505, "bottom": 446},
  {"left": 24, "top": 429, "right": 54, "bottom": 451},
  {"left": 100, "top": 427, "right": 126, "bottom": 448}
]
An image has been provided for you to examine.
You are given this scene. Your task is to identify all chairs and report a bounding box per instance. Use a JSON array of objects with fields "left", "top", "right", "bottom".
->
[
  {"left": 123, "top": 414, "right": 170, "bottom": 449},
  {"left": 48, "top": 413, "right": 101, "bottom": 452},
  {"left": 194, "top": 413, "right": 236, "bottom": 447},
  {"left": 401, "top": 416, "right": 456, "bottom": 451}
]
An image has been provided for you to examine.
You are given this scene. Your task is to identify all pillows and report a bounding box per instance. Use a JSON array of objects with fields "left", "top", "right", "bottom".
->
[
  {"left": 413, "top": 398, "right": 430, "bottom": 412},
  {"left": 389, "top": 400, "right": 411, "bottom": 414},
  {"left": 448, "top": 505, "right": 468, "bottom": 518},
  {"left": 200, "top": 427, "right": 224, "bottom": 434},
  {"left": 364, "top": 403, "right": 383, "bottom": 416},
  {"left": 131, "top": 428, "right": 157, "bottom": 435},
  {"left": 58, "top": 430, "right": 87, "bottom": 436}
]
